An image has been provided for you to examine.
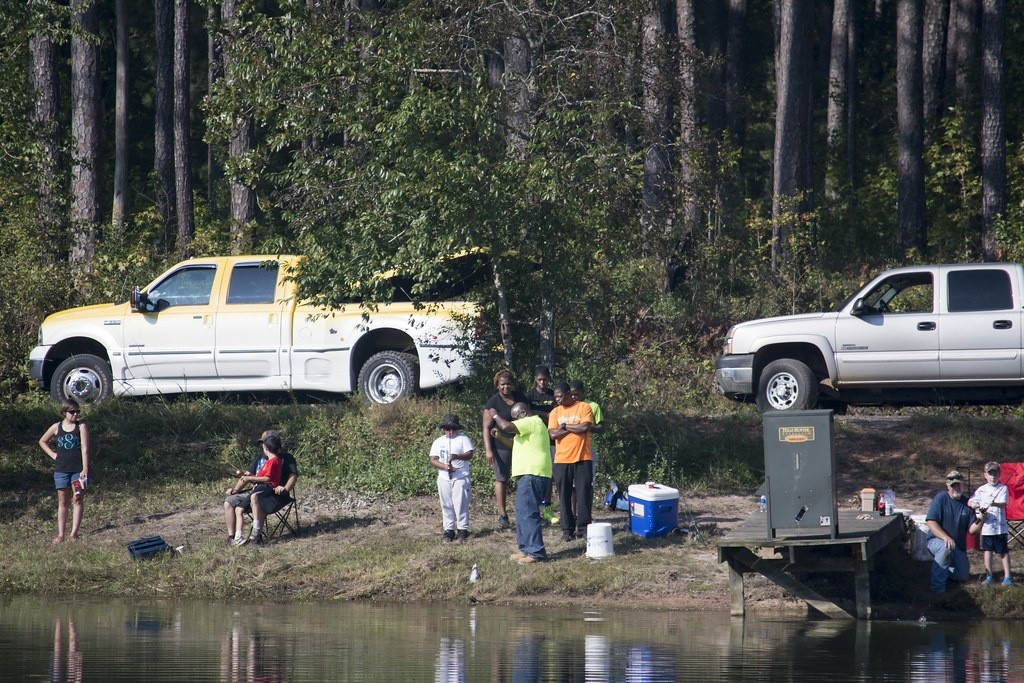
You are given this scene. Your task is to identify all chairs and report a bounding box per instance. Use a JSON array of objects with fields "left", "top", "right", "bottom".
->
[
  {"left": 246, "top": 486, "right": 300, "bottom": 542},
  {"left": 993, "top": 463, "right": 1024, "bottom": 554}
]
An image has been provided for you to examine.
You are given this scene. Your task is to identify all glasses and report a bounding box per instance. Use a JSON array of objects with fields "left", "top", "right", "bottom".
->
[
  {"left": 512, "top": 410, "right": 524, "bottom": 420},
  {"left": 946, "top": 476, "right": 963, "bottom": 480},
  {"left": 444, "top": 426, "right": 459, "bottom": 431},
  {"left": 65, "top": 409, "right": 80, "bottom": 414}
]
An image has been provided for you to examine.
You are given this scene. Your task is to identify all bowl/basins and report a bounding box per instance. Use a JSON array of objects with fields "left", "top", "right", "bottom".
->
[{"left": 646, "top": 482, "right": 655, "bottom": 488}]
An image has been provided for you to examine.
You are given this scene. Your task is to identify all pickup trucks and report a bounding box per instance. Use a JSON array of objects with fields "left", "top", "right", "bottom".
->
[
  {"left": 29, "top": 244, "right": 545, "bottom": 414},
  {"left": 714, "top": 262, "right": 1024, "bottom": 413}
]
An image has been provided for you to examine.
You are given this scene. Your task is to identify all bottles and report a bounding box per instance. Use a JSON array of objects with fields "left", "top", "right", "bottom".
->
[
  {"left": 879, "top": 494, "right": 885, "bottom": 516},
  {"left": 760, "top": 493, "right": 767, "bottom": 513},
  {"left": 471, "top": 563, "right": 480, "bottom": 581}
]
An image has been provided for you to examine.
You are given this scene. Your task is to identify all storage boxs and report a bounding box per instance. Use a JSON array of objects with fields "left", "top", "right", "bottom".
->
[
  {"left": 629, "top": 482, "right": 678, "bottom": 537},
  {"left": 127, "top": 539, "right": 172, "bottom": 560}
]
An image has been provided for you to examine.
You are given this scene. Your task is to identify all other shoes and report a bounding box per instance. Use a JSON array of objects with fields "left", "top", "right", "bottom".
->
[
  {"left": 499, "top": 515, "right": 510, "bottom": 529},
  {"left": 231, "top": 536, "right": 246, "bottom": 549},
  {"left": 561, "top": 534, "right": 575, "bottom": 542},
  {"left": 443, "top": 530, "right": 455, "bottom": 542},
  {"left": 510, "top": 552, "right": 527, "bottom": 561},
  {"left": 982, "top": 575, "right": 994, "bottom": 585},
  {"left": 54, "top": 536, "right": 77, "bottom": 544},
  {"left": 455, "top": 530, "right": 468, "bottom": 540},
  {"left": 222, "top": 536, "right": 234, "bottom": 550},
  {"left": 240, "top": 534, "right": 266, "bottom": 550},
  {"left": 1002, "top": 577, "right": 1013, "bottom": 586},
  {"left": 578, "top": 534, "right": 587, "bottom": 540},
  {"left": 539, "top": 504, "right": 560, "bottom": 524},
  {"left": 930, "top": 600, "right": 952, "bottom": 612},
  {"left": 518, "top": 556, "right": 548, "bottom": 566}
]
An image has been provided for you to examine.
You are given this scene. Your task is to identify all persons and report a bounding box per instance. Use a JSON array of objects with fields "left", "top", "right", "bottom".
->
[
  {"left": 38, "top": 399, "right": 88, "bottom": 544},
  {"left": 925, "top": 470, "right": 987, "bottom": 593},
  {"left": 968, "top": 462, "right": 1011, "bottom": 587},
  {"left": 483, "top": 366, "right": 604, "bottom": 566},
  {"left": 224, "top": 430, "right": 298, "bottom": 546},
  {"left": 231, "top": 436, "right": 282, "bottom": 546},
  {"left": 429, "top": 415, "right": 473, "bottom": 543}
]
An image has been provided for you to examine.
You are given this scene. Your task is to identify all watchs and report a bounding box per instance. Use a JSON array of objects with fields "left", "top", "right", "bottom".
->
[
  {"left": 562, "top": 423, "right": 566, "bottom": 430},
  {"left": 493, "top": 415, "right": 499, "bottom": 420}
]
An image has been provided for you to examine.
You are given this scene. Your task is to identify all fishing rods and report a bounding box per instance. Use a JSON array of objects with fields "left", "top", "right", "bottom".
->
[
  {"left": 611, "top": 398, "right": 706, "bottom": 546},
  {"left": 97, "top": 421, "right": 241, "bottom": 478},
  {"left": 975, "top": 462, "right": 1023, "bottom": 524},
  {"left": 448, "top": 339, "right": 461, "bottom": 480}
]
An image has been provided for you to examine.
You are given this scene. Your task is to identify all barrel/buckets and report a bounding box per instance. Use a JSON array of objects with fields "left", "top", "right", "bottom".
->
[
  {"left": 892, "top": 508, "right": 913, "bottom": 553},
  {"left": 584, "top": 522, "right": 616, "bottom": 560},
  {"left": 909, "top": 514, "right": 934, "bottom": 561}
]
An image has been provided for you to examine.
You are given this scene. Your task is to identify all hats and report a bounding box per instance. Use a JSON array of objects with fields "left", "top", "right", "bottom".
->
[
  {"left": 261, "top": 435, "right": 281, "bottom": 452},
  {"left": 437, "top": 415, "right": 464, "bottom": 429},
  {"left": 984, "top": 461, "right": 1000, "bottom": 472},
  {"left": 946, "top": 470, "right": 964, "bottom": 486},
  {"left": 256, "top": 430, "right": 281, "bottom": 443}
]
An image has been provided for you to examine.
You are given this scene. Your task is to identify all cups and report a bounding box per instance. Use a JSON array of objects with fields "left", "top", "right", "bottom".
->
[
  {"left": 175, "top": 545, "right": 184, "bottom": 556},
  {"left": 79, "top": 478, "right": 87, "bottom": 490},
  {"left": 885, "top": 503, "right": 894, "bottom": 515}
]
